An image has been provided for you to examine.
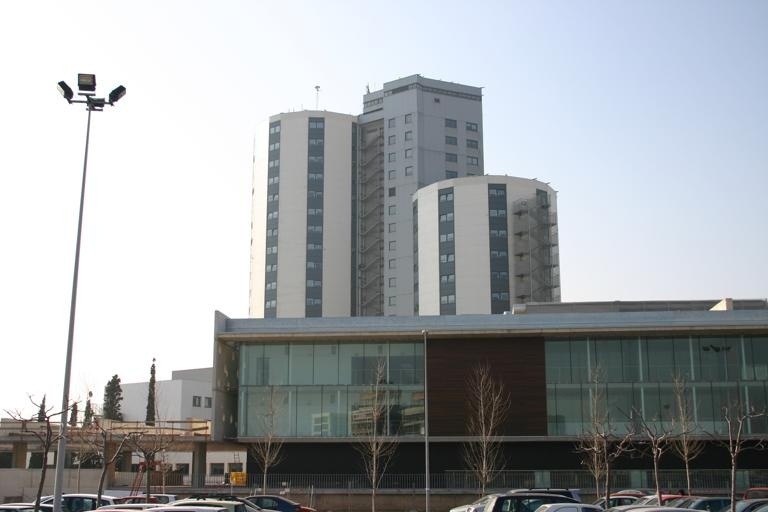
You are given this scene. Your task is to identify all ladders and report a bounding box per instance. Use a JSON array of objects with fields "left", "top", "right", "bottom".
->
[
  {"left": 129, "top": 466, "right": 145, "bottom": 496},
  {"left": 234, "top": 452, "right": 242, "bottom": 473}
]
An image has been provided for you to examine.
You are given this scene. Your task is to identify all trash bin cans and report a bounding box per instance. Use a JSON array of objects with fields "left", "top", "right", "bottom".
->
[{"left": 225, "top": 473, "right": 230, "bottom": 483}]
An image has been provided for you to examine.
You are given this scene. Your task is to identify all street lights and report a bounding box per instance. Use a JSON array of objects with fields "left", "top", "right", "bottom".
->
[{"left": 53, "top": 74, "right": 126, "bottom": 512}]
